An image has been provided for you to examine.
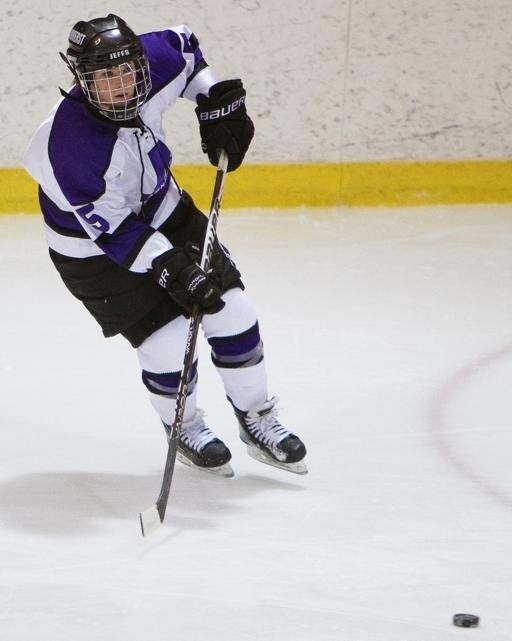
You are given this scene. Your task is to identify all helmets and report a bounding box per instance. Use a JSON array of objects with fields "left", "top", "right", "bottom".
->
[{"left": 65, "top": 15, "right": 151, "bottom": 121}]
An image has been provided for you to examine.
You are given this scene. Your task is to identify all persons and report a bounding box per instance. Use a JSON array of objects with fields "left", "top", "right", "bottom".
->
[{"left": 19, "top": 13, "right": 308, "bottom": 473}]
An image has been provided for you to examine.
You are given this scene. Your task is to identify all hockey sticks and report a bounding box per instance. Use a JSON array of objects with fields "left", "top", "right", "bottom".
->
[{"left": 138, "top": 145, "right": 227, "bottom": 537}]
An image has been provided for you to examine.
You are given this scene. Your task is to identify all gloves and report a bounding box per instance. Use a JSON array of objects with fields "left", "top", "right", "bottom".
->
[
  {"left": 145, "top": 248, "right": 225, "bottom": 318},
  {"left": 194, "top": 79, "right": 255, "bottom": 172}
]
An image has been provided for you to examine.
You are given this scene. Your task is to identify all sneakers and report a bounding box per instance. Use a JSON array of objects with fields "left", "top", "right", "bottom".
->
[
  {"left": 162, "top": 410, "right": 231, "bottom": 468},
  {"left": 226, "top": 396, "right": 306, "bottom": 462}
]
